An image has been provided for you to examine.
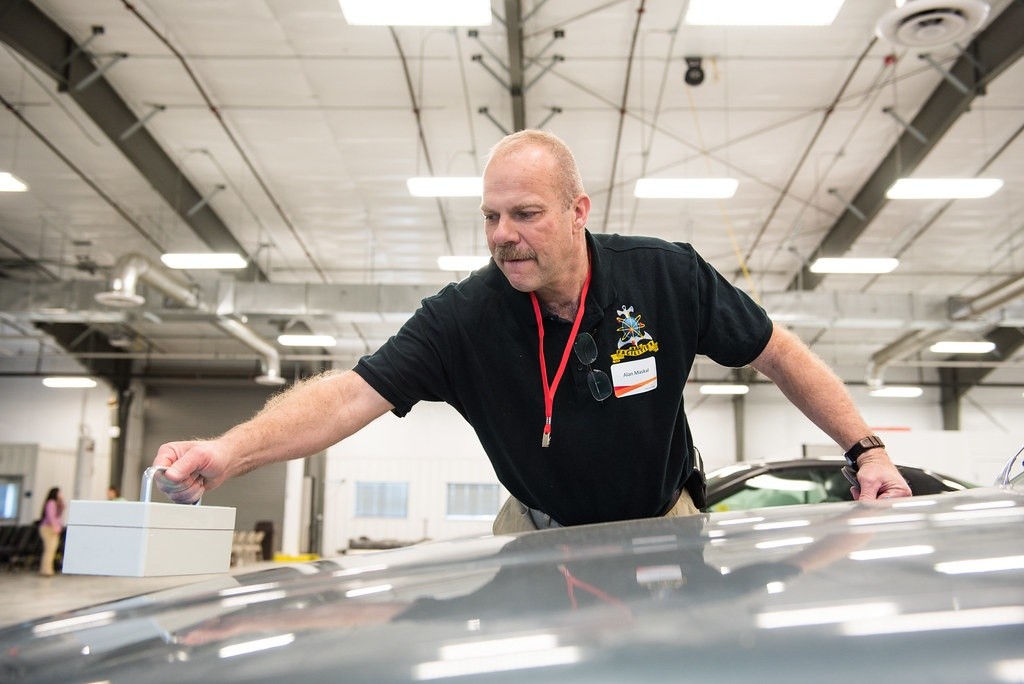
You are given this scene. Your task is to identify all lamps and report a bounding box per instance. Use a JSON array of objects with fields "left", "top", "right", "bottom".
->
[{"left": 930, "top": 331, "right": 996, "bottom": 354}]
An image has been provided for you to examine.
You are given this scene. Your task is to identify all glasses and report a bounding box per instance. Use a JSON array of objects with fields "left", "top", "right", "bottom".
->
[{"left": 572, "top": 332, "right": 613, "bottom": 402}]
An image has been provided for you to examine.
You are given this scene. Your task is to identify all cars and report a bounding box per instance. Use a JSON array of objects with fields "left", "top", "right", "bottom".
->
[{"left": 706, "top": 454, "right": 984, "bottom": 514}]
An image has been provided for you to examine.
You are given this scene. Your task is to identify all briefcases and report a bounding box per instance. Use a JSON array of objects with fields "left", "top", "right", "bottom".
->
[{"left": 61, "top": 465, "right": 236, "bottom": 576}]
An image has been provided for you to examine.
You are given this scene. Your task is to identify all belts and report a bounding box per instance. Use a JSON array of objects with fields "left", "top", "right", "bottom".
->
[{"left": 519, "top": 485, "right": 682, "bottom": 530}]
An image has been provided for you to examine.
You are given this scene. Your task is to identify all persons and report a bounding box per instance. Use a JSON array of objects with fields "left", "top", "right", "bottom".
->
[
  {"left": 153, "top": 130, "right": 914, "bottom": 504},
  {"left": 38, "top": 486, "right": 64, "bottom": 577},
  {"left": 106, "top": 486, "right": 117, "bottom": 500}
]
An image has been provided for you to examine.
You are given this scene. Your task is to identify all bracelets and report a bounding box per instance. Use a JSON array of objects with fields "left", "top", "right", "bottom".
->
[{"left": 843, "top": 434, "right": 885, "bottom": 470}]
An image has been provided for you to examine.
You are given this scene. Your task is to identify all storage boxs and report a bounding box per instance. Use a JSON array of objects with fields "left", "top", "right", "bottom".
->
[{"left": 61, "top": 499, "right": 237, "bottom": 577}]
